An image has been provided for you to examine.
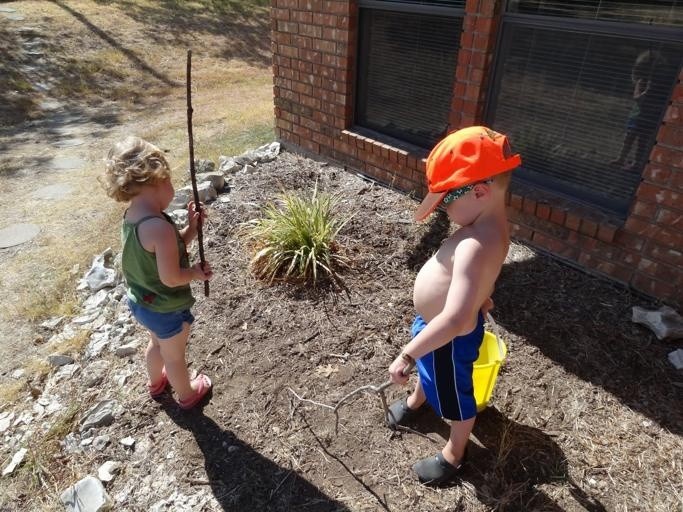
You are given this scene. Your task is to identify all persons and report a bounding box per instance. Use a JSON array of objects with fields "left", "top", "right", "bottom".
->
[
  {"left": 382, "top": 125, "right": 523, "bottom": 484},
  {"left": 609, "top": 57, "right": 668, "bottom": 174},
  {"left": 96, "top": 136, "right": 212, "bottom": 408}
]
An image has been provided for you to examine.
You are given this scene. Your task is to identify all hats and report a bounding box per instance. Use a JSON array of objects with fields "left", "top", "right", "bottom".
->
[{"left": 414, "top": 124, "right": 521, "bottom": 220}]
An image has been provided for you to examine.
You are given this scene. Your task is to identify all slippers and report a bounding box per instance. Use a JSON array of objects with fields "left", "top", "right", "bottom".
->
[
  {"left": 415, "top": 451, "right": 470, "bottom": 483},
  {"left": 148, "top": 367, "right": 168, "bottom": 395},
  {"left": 385, "top": 396, "right": 428, "bottom": 425},
  {"left": 177, "top": 374, "right": 212, "bottom": 409}
]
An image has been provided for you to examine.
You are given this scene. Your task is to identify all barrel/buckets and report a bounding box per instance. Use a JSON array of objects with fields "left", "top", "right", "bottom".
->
[{"left": 472, "top": 312, "right": 508, "bottom": 414}]
